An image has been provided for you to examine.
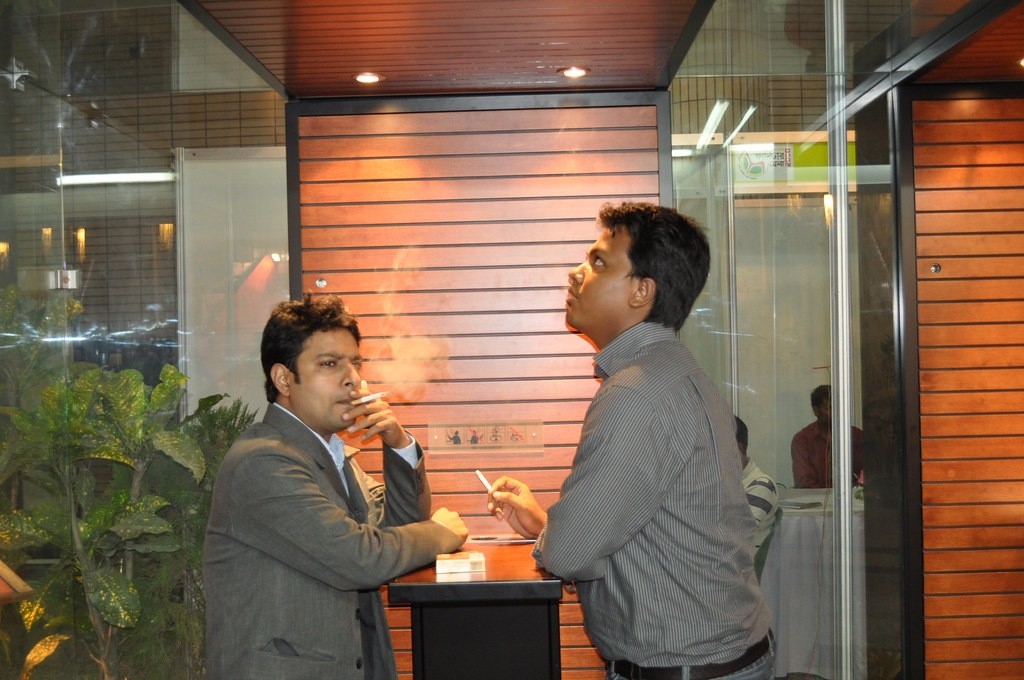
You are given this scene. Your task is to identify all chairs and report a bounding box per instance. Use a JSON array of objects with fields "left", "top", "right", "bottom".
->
[{"left": 753, "top": 507, "right": 783, "bottom": 585}]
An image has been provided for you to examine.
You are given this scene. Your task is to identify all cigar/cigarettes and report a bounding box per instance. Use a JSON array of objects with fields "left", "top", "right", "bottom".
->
[
  {"left": 476, "top": 469, "right": 491, "bottom": 490},
  {"left": 351, "top": 391, "right": 384, "bottom": 405}
]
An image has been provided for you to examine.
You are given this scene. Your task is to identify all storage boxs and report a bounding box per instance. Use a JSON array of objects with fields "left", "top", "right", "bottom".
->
[{"left": 436, "top": 553, "right": 485, "bottom": 573}]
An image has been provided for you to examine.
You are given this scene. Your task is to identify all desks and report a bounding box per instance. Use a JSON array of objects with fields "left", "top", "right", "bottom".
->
[{"left": 759, "top": 485, "right": 867, "bottom": 680}]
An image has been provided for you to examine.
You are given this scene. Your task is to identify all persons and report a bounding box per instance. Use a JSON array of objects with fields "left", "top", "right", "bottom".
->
[
  {"left": 204, "top": 291, "right": 469, "bottom": 680},
  {"left": 487, "top": 203, "right": 776, "bottom": 680},
  {"left": 791, "top": 385, "right": 865, "bottom": 488},
  {"left": 735, "top": 416, "right": 780, "bottom": 562}
]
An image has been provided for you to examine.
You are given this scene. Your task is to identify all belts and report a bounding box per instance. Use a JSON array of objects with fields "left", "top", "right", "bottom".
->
[{"left": 605, "top": 628, "right": 774, "bottom": 680}]
